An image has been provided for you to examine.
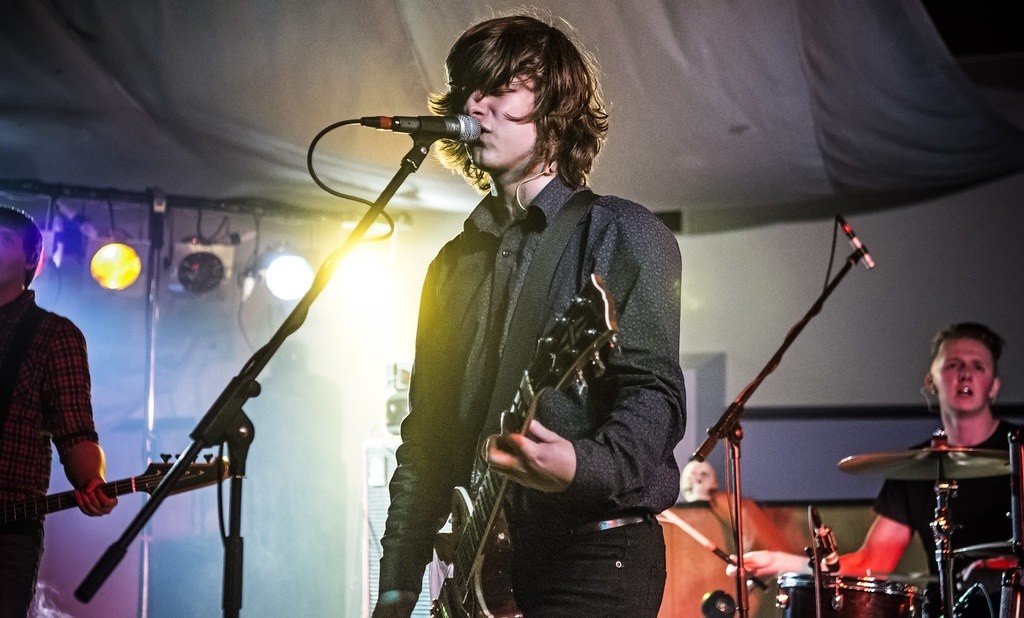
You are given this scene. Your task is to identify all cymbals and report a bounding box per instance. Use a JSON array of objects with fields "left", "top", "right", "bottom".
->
[
  {"left": 834, "top": 427, "right": 1013, "bottom": 481},
  {"left": 952, "top": 540, "right": 1014, "bottom": 558}
]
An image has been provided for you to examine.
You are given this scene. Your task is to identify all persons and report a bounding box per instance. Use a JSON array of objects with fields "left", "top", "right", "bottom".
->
[
  {"left": 0, "top": 205, "right": 118, "bottom": 618},
  {"left": 730, "top": 320, "right": 1024, "bottom": 618},
  {"left": 370, "top": 14, "right": 688, "bottom": 618}
]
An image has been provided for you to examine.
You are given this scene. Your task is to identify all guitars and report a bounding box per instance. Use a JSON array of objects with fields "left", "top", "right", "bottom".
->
[
  {"left": 0, "top": 449, "right": 233, "bottom": 527},
  {"left": 428, "top": 268, "right": 624, "bottom": 618}
]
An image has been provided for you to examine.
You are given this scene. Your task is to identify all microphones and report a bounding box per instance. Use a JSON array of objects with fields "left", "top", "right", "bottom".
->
[
  {"left": 360, "top": 113, "right": 482, "bottom": 145},
  {"left": 841, "top": 222, "right": 877, "bottom": 271},
  {"left": 815, "top": 518, "right": 840, "bottom": 572}
]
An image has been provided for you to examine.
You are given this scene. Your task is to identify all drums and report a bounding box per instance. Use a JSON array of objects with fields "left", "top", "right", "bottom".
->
[{"left": 775, "top": 570, "right": 927, "bottom": 618}]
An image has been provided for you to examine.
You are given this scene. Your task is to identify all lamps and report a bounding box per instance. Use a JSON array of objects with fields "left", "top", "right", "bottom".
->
[
  {"left": 85, "top": 238, "right": 152, "bottom": 300},
  {"left": 38, "top": 228, "right": 80, "bottom": 261},
  {"left": 259, "top": 245, "right": 314, "bottom": 299},
  {"left": 171, "top": 242, "right": 235, "bottom": 296}
]
